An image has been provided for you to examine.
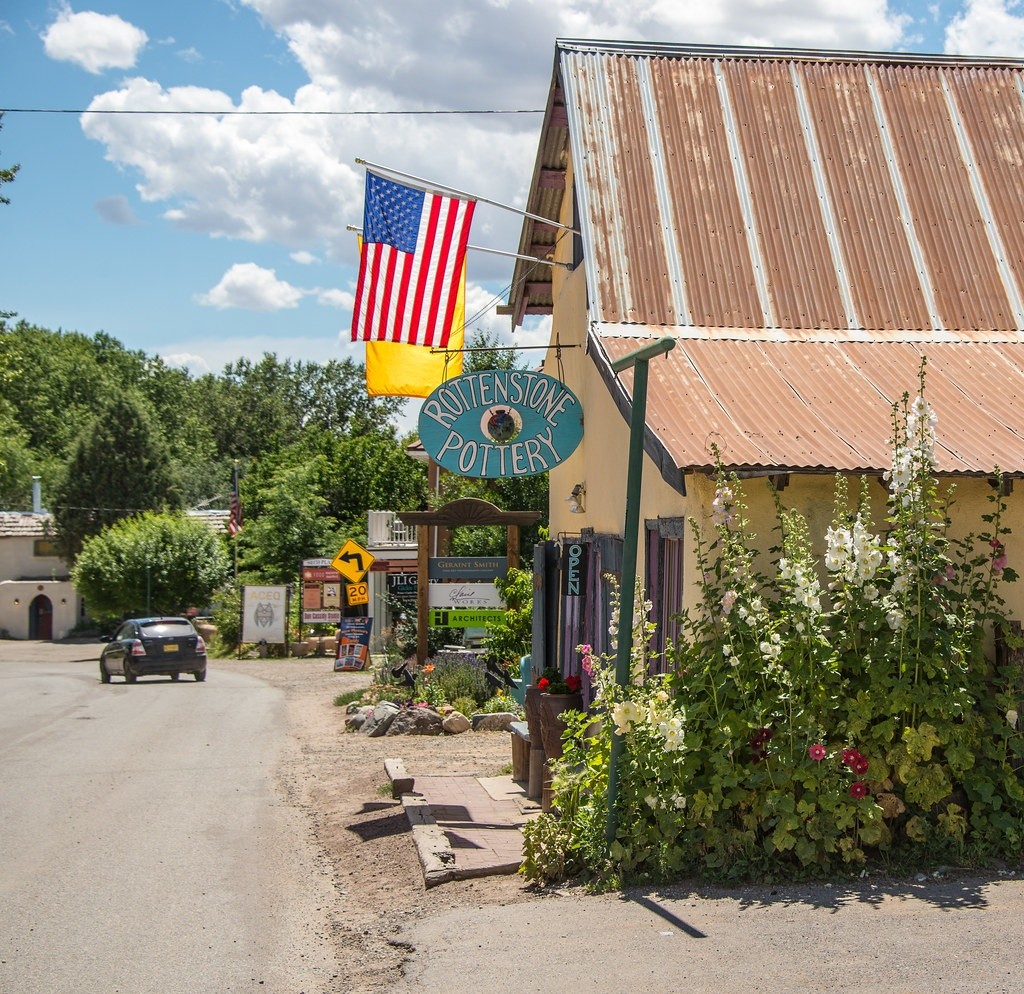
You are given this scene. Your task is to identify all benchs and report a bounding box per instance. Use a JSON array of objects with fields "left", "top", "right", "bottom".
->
[{"left": 508, "top": 721, "right": 531, "bottom": 781}]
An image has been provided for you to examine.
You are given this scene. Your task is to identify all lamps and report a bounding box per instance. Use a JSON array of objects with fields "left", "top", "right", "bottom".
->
[
  {"left": 565, "top": 484, "right": 586, "bottom": 513},
  {"left": 554, "top": 527, "right": 593, "bottom": 548}
]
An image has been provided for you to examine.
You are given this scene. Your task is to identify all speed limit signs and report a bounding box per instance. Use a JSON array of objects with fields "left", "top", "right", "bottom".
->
[{"left": 346, "top": 582, "right": 369, "bottom": 606}]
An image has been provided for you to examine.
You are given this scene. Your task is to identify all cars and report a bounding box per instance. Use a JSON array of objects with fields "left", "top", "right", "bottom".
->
[{"left": 99, "top": 617, "right": 208, "bottom": 683}]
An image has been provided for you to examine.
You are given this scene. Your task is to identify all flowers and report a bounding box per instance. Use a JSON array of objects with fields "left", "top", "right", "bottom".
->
[{"left": 536, "top": 665, "right": 583, "bottom": 695}]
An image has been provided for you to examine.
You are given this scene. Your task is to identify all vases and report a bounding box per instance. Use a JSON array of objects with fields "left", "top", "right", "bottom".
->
[
  {"left": 526, "top": 685, "right": 546, "bottom": 751},
  {"left": 539, "top": 693, "right": 583, "bottom": 762}
]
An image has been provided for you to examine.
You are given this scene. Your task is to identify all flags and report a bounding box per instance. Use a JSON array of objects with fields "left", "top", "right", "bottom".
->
[
  {"left": 355, "top": 235, "right": 468, "bottom": 401},
  {"left": 226, "top": 469, "right": 244, "bottom": 533},
  {"left": 351, "top": 170, "right": 477, "bottom": 350}
]
